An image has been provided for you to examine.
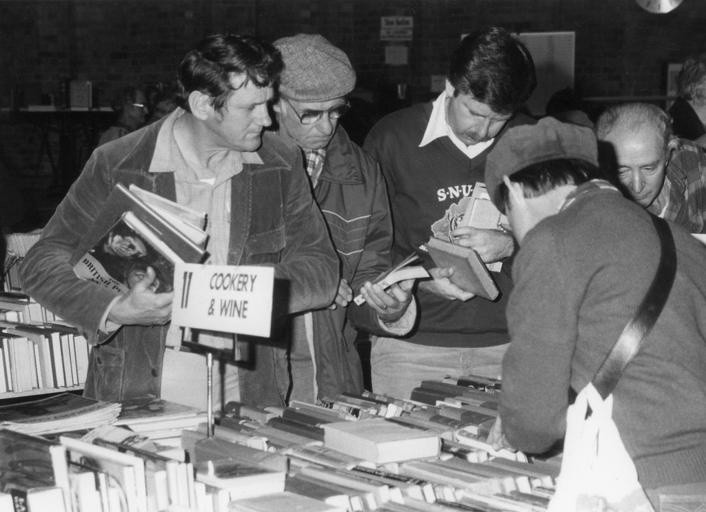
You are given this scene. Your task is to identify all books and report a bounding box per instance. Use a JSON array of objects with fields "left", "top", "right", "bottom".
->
[
  {"left": 454, "top": 183, "right": 510, "bottom": 272},
  {"left": 424, "top": 237, "right": 502, "bottom": 303},
  {"left": 70, "top": 179, "right": 211, "bottom": 315},
  {"left": 0, "top": 225, "right": 91, "bottom": 391},
  {"left": 0, "top": 373, "right": 569, "bottom": 512},
  {"left": 350, "top": 250, "right": 431, "bottom": 308}
]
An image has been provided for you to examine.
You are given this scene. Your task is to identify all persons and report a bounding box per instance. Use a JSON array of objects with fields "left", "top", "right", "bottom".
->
[
  {"left": 151, "top": 89, "right": 178, "bottom": 123},
  {"left": 358, "top": 24, "right": 567, "bottom": 396},
  {"left": 96, "top": 84, "right": 149, "bottom": 149},
  {"left": 254, "top": 30, "right": 403, "bottom": 411},
  {"left": 21, "top": 28, "right": 343, "bottom": 415},
  {"left": 598, "top": 102, "right": 705, "bottom": 244},
  {"left": 667, "top": 54, "right": 706, "bottom": 141},
  {"left": 481, "top": 115, "right": 706, "bottom": 512}
]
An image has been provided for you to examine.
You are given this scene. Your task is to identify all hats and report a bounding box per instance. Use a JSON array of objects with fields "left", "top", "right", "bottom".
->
[
  {"left": 266, "top": 31, "right": 357, "bottom": 103},
  {"left": 483, "top": 116, "right": 601, "bottom": 215}
]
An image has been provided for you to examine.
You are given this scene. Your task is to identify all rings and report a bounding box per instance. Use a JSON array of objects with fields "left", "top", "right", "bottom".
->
[{"left": 380, "top": 304, "right": 387, "bottom": 309}]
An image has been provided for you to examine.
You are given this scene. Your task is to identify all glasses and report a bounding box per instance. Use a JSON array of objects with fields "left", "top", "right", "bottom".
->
[
  {"left": 276, "top": 93, "right": 352, "bottom": 126},
  {"left": 497, "top": 205, "right": 514, "bottom": 235}
]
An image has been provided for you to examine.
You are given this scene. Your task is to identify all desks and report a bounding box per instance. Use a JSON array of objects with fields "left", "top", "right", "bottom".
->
[{"left": 0, "top": 105, "right": 121, "bottom": 183}]
